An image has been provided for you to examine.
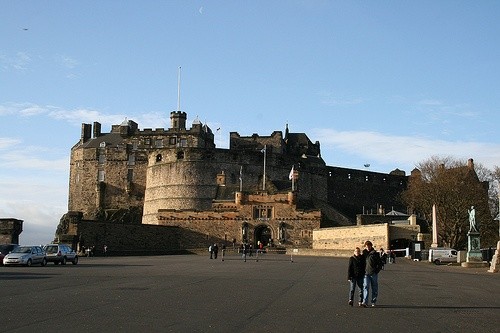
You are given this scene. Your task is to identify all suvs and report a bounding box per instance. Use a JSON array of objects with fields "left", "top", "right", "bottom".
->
[{"left": 43, "top": 242, "right": 78, "bottom": 265}]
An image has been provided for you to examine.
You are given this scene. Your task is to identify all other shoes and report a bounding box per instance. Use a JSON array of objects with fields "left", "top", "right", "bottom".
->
[
  {"left": 358, "top": 302, "right": 362, "bottom": 307},
  {"left": 362, "top": 302, "right": 368, "bottom": 308},
  {"left": 348, "top": 300, "right": 353, "bottom": 306},
  {"left": 371, "top": 303, "right": 376, "bottom": 307}
]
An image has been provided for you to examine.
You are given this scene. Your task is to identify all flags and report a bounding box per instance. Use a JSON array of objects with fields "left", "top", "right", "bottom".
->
[
  {"left": 261, "top": 147, "right": 266, "bottom": 153},
  {"left": 240, "top": 169, "right": 242, "bottom": 178},
  {"left": 288, "top": 168, "right": 294, "bottom": 180}
]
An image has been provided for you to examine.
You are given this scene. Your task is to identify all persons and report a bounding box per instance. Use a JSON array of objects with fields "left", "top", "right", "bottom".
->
[
  {"left": 347, "top": 240, "right": 387, "bottom": 307},
  {"left": 249, "top": 241, "right": 264, "bottom": 256},
  {"left": 208, "top": 243, "right": 218, "bottom": 259},
  {"left": 468, "top": 206, "right": 479, "bottom": 232},
  {"left": 81, "top": 245, "right": 109, "bottom": 256},
  {"left": 390, "top": 250, "right": 396, "bottom": 263}
]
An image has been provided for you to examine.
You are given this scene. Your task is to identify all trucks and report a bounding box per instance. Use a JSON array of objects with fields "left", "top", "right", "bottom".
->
[{"left": 428, "top": 248, "right": 458, "bottom": 265}]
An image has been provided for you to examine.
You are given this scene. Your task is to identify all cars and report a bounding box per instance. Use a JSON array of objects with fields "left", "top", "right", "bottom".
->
[{"left": 0, "top": 243, "right": 47, "bottom": 267}]
